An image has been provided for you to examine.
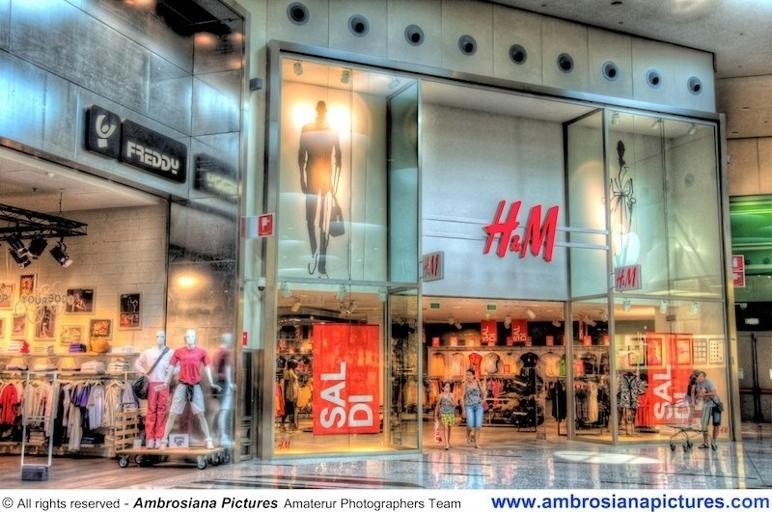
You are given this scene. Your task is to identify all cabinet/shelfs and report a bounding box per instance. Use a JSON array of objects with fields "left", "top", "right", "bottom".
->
[{"left": 0, "top": 351, "right": 142, "bottom": 375}]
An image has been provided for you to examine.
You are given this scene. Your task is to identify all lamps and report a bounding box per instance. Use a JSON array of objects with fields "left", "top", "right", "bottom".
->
[
  {"left": 4, "top": 219, "right": 74, "bottom": 271},
  {"left": 281, "top": 283, "right": 706, "bottom": 334},
  {"left": 292, "top": 59, "right": 350, "bottom": 85},
  {"left": 610, "top": 109, "right": 698, "bottom": 136}
]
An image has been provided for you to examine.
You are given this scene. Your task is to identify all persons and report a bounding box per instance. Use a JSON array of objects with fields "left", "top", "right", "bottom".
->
[
  {"left": 211, "top": 333, "right": 235, "bottom": 446},
  {"left": 153, "top": 329, "right": 222, "bottom": 451},
  {"left": 693, "top": 370, "right": 723, "bottom": 450},
  {"left": 460, "top": 368, "right": 486, "bottom": 449},
  {"left": 279, "top": 359, "right": 299, "bottom": 431},
  {"left": 602, "top": 140, "right": 642, "bottom": 292},
  {"left": 73, "top": 292, "right": 87, "bottom": 313},
  {"left": 687, "top": 370, "right": 700, "bottom": 429},
  {"left": 435, "top": 382, "right": 459, "bottom": 451},
  {"left": 298, "top": 100, "right": 341, "bottom": 280},
  {"left": 134, "top": 331, "right": 180, "bottom": 449}
]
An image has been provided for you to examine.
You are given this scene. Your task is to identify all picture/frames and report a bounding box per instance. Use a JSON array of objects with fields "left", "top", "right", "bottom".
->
[
  {"left": 57, "top": 324, "right": 84, "bottom": 348},
  {"left": 0, "top": 281, "right": 18, "bottom": 309},
  {"left": 9, "top": 312, "right": 30, "bottom": 342},
  {"left": 116, "top": 290, "right": 145, "bottom": 331},
  {"left": 61, "top": 286, "right": 98, "bottom": 315},
  {"left": 87, "top": 316, "right": 116, "bottom": 341},
  {"left": 17, "top": 271, "right": 39, "bottom": 300},
  {"left": 32, "top": 302, "right": 58, "bottom": 343},
  {"left": 623, "top": 336, "right": 726, "bottom": 372}
]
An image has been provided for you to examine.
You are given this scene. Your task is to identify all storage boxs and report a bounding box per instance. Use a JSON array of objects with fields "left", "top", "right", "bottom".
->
[{"left": 168, "top": 428, "right": 192, "bottom": 449}]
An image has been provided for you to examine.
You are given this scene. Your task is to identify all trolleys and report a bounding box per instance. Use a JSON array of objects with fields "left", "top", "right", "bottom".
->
[
  {"left": 662, "top": 397, "right": 719, "bottom": 452},
  {"left": 110, "top": 371, "right": 231, "bottom": 468}
]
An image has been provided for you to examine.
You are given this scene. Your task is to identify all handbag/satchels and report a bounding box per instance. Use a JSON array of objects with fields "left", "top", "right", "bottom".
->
[
  {"left": 707, "top": 396, "right": 725, "bottom": 412},
  {"left": 432, "top": 420, "right": 443, "bottom": 444},
  {"left": 131, "top": 347, "right": 169, "bottom": 399},
  {"left": 475, "top": 382, "right": 489, "bottom": 411},
  {"left": 329, "top": 196, "right": 346, "bottom": 238}
]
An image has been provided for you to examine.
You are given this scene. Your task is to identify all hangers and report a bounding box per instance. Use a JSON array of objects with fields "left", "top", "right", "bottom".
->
[{"left": 2, "top": 372, "right": 123, "bottom": 392}]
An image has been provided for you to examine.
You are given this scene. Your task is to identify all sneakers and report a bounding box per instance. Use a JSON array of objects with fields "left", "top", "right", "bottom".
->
[
  {"left": 698, "top": 442, "right": 719, "bottom": 451},
  {"left": 444, "top": 439, "right": 482, "bottom": 451}
]
